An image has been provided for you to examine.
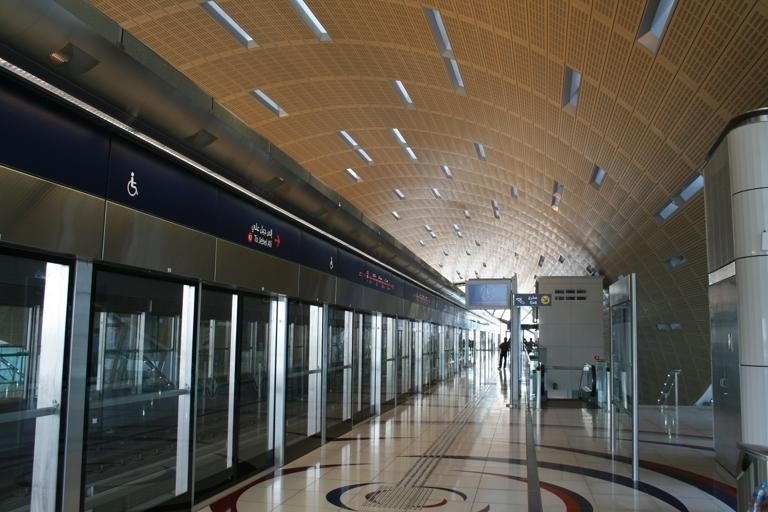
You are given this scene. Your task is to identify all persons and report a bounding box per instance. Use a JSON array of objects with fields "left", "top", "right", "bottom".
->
[{"left": 498, "top": 337, "right": 511, "bottom": 368}]
